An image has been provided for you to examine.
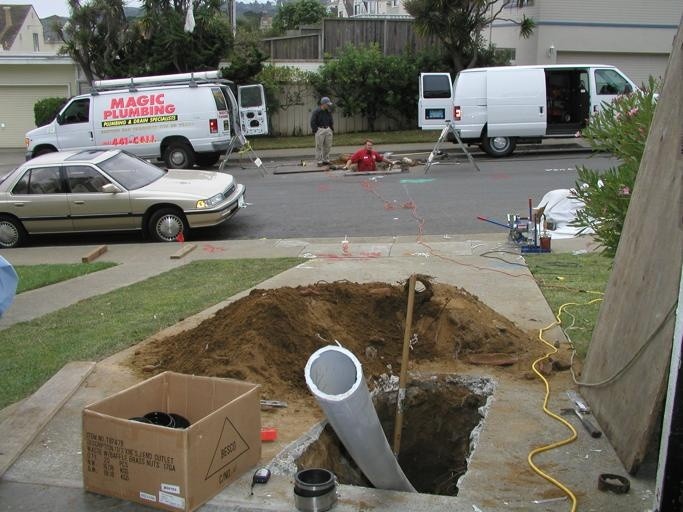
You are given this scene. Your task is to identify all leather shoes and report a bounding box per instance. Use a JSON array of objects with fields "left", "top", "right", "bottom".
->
[
  {"left": 317, "top": 163, "right": 321, "bottom": 167},
  {"left": 323, "top": 162, "right": 332, "bottom": 165}
]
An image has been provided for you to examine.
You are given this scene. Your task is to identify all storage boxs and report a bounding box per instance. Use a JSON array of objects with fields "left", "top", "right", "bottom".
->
[{"left": 79, "top": 369, "right": 264, "bottom": 511}]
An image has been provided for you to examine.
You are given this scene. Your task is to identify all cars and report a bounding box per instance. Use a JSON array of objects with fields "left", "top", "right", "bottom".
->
[{"left": 0, "top": 153, "right": 247, "bottom": 244}]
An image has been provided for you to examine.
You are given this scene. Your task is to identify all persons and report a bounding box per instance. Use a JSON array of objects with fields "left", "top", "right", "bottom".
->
[
  {"left": 341, "top": 138, "right": 395, "bottom": 173},
  {"left": 310, "top": 95, "right": 335, "bottom": 168}
]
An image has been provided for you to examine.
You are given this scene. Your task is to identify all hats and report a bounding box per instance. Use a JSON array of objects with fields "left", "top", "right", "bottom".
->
[{"left": 320, "top": 97, "right": 332, "bottom": 106}]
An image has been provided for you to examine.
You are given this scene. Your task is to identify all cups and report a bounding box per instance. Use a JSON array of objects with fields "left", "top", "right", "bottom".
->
[{"left": 341, "top": 240, "right": 350, "bottom": 255}]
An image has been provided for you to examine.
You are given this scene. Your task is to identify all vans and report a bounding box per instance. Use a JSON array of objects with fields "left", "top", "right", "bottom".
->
[
  {"left": 24, "top": 72, "right": 266, "bottom": 164},
  {"left": 414, "top": 63, "right": 659, "bottom": 159}
]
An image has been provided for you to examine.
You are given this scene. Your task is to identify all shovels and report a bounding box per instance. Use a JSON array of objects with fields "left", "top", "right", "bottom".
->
[{"left": 558, "top": 390, "right": 601, "bottom": 437}]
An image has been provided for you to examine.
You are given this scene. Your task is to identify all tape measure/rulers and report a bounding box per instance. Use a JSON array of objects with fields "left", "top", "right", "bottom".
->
[{"left": 251, "top": 468, "right": 271, "bottom": 495}]
